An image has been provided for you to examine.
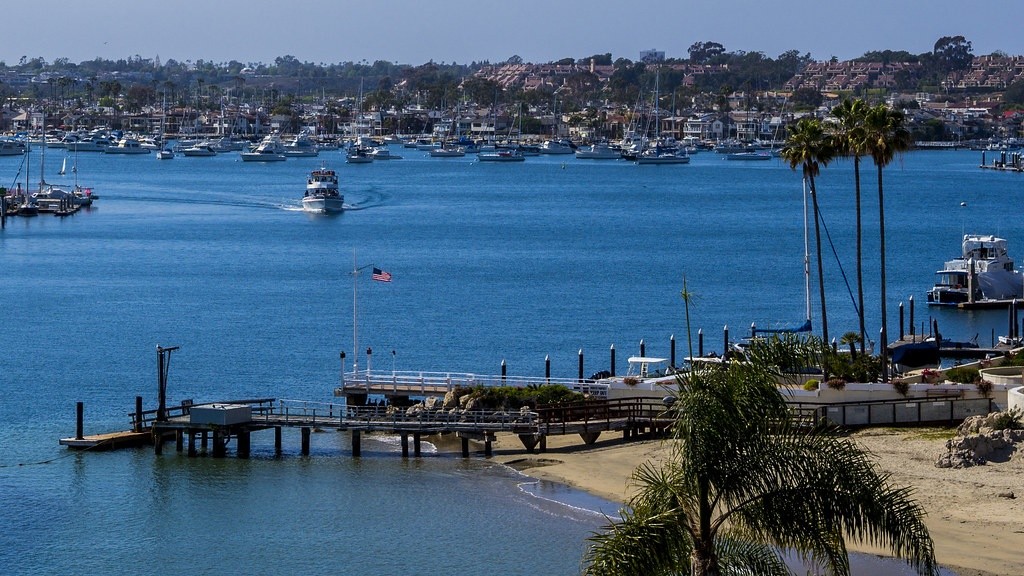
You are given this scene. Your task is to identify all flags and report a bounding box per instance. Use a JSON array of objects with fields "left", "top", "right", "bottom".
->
[{"left": 372, "top": 268, "right": 391, "bottom": 282}]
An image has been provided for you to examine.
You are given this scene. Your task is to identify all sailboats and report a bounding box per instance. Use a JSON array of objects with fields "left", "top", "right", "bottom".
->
[
  {"left": 733, "top": 176, "right": 875, "bottom": 364},
  {"left": 5, "top": 111, "right": 97, "bottom": 206},
  {"left": 0, "top": 71, "right": 787, "bottom": 164}
]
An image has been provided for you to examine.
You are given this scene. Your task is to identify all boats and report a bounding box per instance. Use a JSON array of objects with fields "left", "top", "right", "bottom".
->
[
  {"left": 593, "top": 356, "right": 713, "bottom": 388},
  {"left": 925, "top": 234, "right": 1024, "bottom": 306},
  {"left": 300, "top": 159, "right": 345, "bottom": 211}
]
